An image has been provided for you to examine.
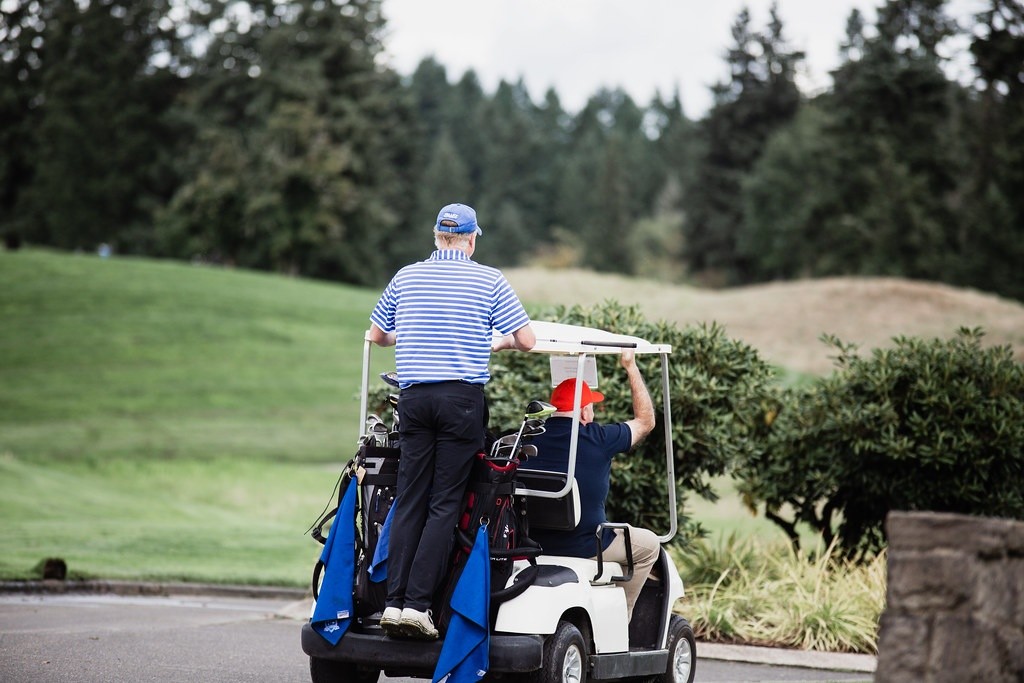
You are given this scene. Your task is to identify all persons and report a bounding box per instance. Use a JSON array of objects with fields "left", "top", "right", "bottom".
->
[
  {"left": 515, "top": 347, "right": 659, "bottom": 625},
  {"left": 368, "top": 203, "right": 538, "bottom": 643}
]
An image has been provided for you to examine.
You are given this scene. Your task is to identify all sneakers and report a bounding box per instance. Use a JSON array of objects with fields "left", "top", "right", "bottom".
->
[
  {"left": 380, "top": 605, "right": 402, "bottom": 638},
  {"left": 399, "top": 607, "right": 438, "bottom": 640}
]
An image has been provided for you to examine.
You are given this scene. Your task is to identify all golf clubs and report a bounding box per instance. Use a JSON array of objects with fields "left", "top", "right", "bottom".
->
[
  {"left": 488, "top": 400, "right": 558, "bottom": 472},
  {"left": 362, "top": 371, "right": 402, "bottom": 449}
]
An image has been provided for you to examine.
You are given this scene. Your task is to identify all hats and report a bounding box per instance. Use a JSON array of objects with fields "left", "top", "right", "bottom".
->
[
  {"left": 551, "top": 379, "right": 604, "bottom": 411},
  {"left": 437, "top": 203, "right": 483, "bottom": 237}
]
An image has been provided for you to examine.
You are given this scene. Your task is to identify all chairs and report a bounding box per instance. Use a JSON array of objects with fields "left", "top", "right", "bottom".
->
[{"left": 516, "top": 468, "right": 634, "bottom": 585}]
